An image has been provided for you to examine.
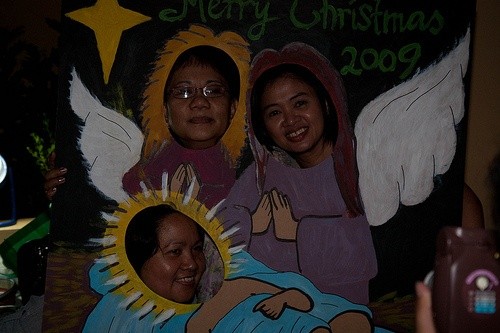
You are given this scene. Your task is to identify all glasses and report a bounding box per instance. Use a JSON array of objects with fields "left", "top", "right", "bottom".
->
[{"left": 170, "top": 84, "right": 229, "bottom": 99}]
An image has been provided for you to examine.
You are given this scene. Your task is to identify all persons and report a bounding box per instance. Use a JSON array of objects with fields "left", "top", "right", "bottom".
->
[
  {"left": 125, "top": 204, "right": 207, "bottom": 305},
  {"left": 42, "top": 50, "right": 240, "bottom": 203},
  {"left": 251, "top": 64, "right": 339, "bottom": 170}
]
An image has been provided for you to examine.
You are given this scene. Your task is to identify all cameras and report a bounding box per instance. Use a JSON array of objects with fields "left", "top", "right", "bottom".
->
[{"left": 432, "top": 226, "right": 500, "bottom": 333}]
